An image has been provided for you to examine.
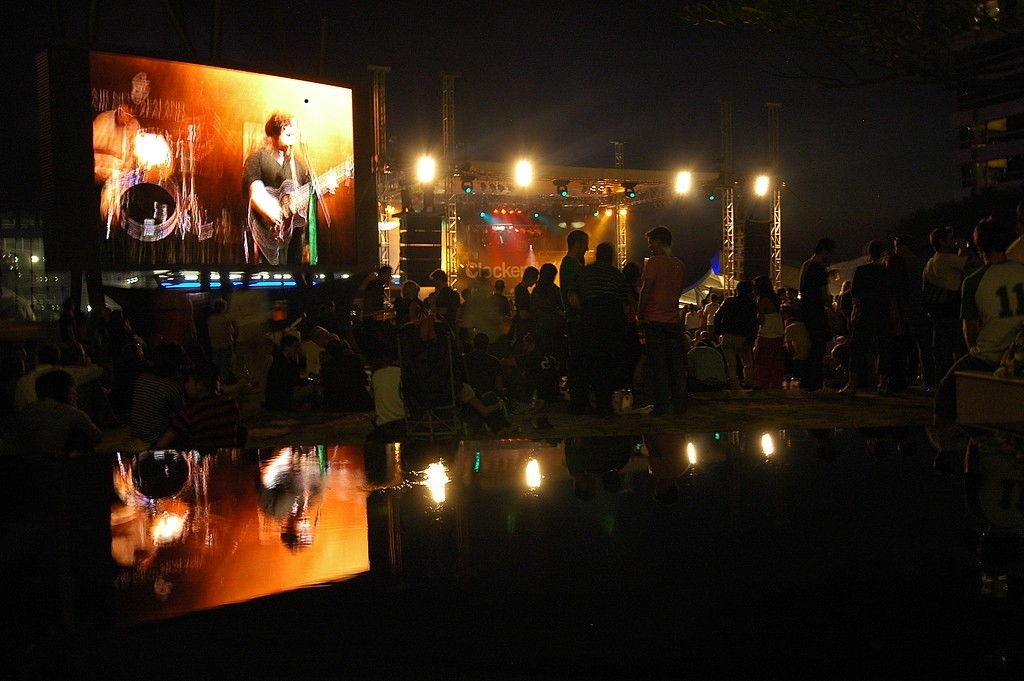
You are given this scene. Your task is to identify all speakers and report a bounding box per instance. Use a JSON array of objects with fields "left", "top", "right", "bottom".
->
[
  {"left": 732, "top": 219, "right": 772, "bottom": 281},
  {"left": 398, "top": 212, "right": 441, "bottom": 287}
]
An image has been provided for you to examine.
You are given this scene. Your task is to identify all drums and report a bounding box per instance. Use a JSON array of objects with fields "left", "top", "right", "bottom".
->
[
  {"left": 114, "top": 169, "right": 181, "bottom": 243},
  {"left": 127, "top": 449, "right": 190, "bottom": 500}
]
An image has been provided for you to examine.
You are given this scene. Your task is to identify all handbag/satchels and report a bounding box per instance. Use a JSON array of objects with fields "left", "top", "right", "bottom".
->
[
  {"left": 612, "top": 388, "right": 632, "bottom": 415},
  {"left": 848, "top": 300, "right": 865, "bottom": 324}
]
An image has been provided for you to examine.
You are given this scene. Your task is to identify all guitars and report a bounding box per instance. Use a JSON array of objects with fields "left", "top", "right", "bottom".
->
[{"left": 248, "top": 156, "right": 354, "bottom": 250}]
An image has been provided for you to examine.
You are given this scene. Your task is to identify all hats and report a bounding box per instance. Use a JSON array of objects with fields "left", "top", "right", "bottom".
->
[
  {"left": 700, "top": 331, "right": 710, "bottom": 339},
  {"left": 930, "top": 226, "right": 953, "bottom": 243}
]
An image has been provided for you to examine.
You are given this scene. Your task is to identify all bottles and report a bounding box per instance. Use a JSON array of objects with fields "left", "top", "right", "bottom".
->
[{"left": 612, "top": 389, "right": 633, "bottom": 414}]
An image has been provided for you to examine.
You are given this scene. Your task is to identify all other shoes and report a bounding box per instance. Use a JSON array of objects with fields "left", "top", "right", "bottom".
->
[
  {"left": 731, "top": 379, "right": 739, "bottom": 388},
  {"left": 881, "top": 385, "right": 907, "bottom": 394},
  {"left": 744, "top": 383, "right": 762, "bottom": 390},
  {"left": 838, "top": 384, "right": 856, "bottom": 395},
  {"left": 918, "top": 388, "right": 935, "bottom": 396},
  {"left": 532, "top": 416, "right": 553, "bottom": 433},
  {"left": 110, "top": 420, "right": 127, "bottom": 427},
  {"left": 808, "top": 387, "right": 833, "bottom": 394}
]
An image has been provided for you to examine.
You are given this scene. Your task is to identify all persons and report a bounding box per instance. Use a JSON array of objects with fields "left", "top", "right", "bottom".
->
[
  {"left": 0, "top": 200, "right": 1024, "bottom": 459},
  {"left": 565, "top": 425, "right": 1024, "bottom": 597},
  {"left": 257, "top": 445, "right": 331, "bottom": 552},
  {"left": 94, "top": 98, "right": 141, "bottom": 200},
  {"left": 113, "top": 456, "right": 160, "bottom": 582},
  {"left": 243, "top": 111, "right": 339, "bottom": 264}
]
